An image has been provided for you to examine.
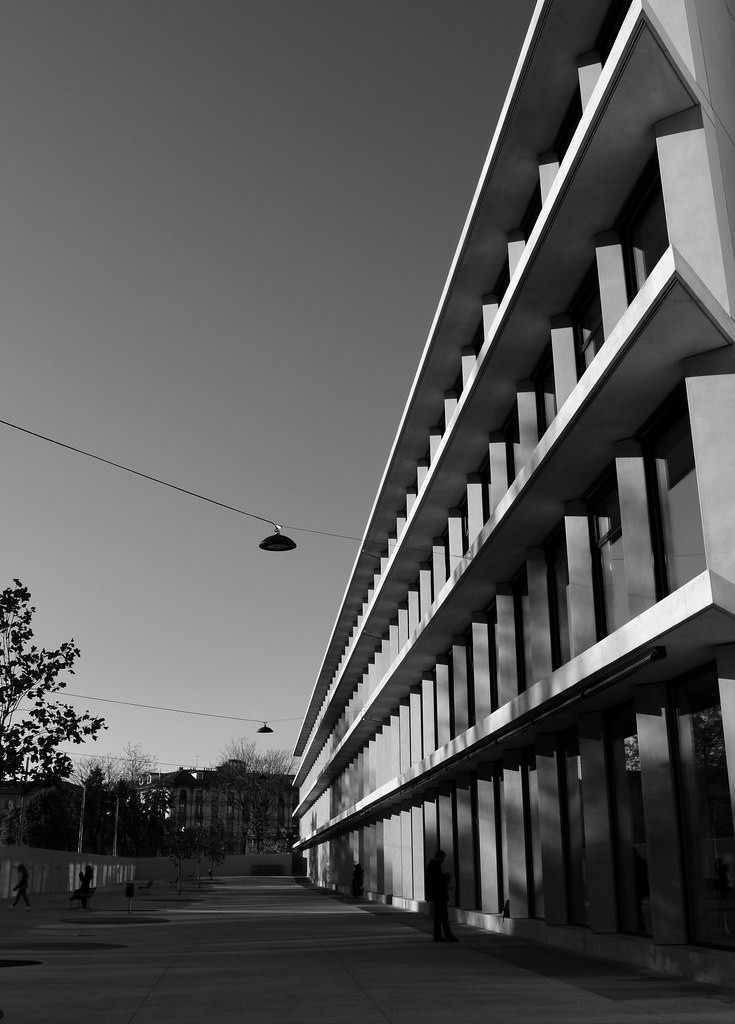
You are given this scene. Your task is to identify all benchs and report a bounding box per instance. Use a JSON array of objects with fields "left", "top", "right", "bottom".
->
[
  {"left": 68, "top": 887, "right": 96, "bottom": 911},
  {"left": 169, "top": 876, "right": 179, "bottom": 888},
  {"left": 137, "top": 881, "right": 153, "bottom": 896}
]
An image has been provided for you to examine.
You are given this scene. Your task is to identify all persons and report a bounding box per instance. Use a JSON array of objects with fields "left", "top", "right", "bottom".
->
[
  {"left": 79, "top": 865, "right": 93, "bottom": 911},
  {"left": 352, "top": 863, "right": 364, "bottom": 897},
  {"left": 8, "top": 864, "right": 32, "bottom": 911},
  {"left": 425, "top": 850, "right": 460, "bottom": 943}
]
